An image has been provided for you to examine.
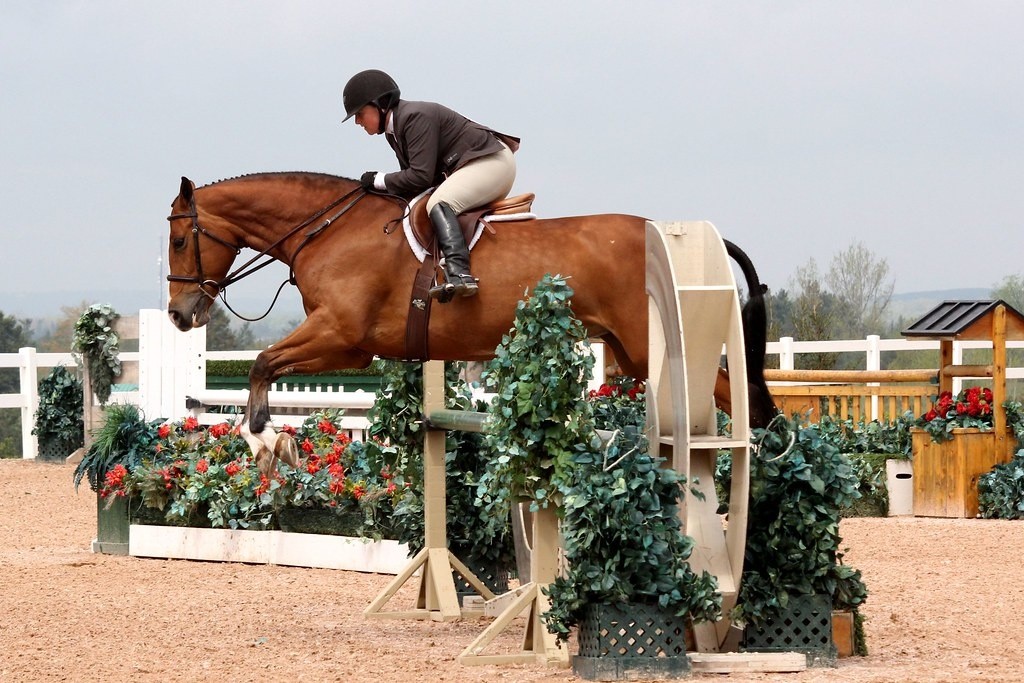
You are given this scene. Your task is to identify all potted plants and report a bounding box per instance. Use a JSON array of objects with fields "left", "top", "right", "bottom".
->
[
  {"left": 30, "top": 366, "right": 84, "bottom": 464},
  {"left": 369, "top": 272, "right": 872, "bottom": 683}
]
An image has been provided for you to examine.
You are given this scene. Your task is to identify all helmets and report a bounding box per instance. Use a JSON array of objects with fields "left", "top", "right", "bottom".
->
[{"left": 341, "top": 69, "right": 400, "bottom": 123}]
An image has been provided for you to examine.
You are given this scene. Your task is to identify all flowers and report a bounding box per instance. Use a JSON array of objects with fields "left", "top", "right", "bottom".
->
[
  {"left": 916, "top": 388, "right": 1024, "bottom": 444},
  {"left": 99, "top": 417, "right": 408, "bottom": 540}
]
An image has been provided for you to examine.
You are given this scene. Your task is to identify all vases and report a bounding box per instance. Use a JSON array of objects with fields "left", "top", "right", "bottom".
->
[{"left": 127, "top": 524, "right": 422, "bottom": 577}]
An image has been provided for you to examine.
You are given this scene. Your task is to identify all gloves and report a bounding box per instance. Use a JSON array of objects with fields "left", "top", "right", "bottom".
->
[{"left": 360, "top": 172, "right": 378, "bottom": 190}]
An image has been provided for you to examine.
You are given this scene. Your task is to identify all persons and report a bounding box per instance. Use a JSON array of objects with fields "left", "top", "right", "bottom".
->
[{"left": 341, "top": 70, "right": 520, "bottom": 300}]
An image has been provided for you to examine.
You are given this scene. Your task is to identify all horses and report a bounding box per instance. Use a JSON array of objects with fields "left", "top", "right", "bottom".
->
[{"left": 166, "top": 170, "right": 791, "bottom": 482}]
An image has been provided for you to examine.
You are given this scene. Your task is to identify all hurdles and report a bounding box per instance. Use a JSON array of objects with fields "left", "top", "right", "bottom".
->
[
  {"left": 762, "top": 295, "right": 1024, "bottom": 525},
  {"left": 126, "top": 300, "right": 500, "bottom": 581},
  {"left": 355, "top": 355, "right": 576, "bottom": 674}
]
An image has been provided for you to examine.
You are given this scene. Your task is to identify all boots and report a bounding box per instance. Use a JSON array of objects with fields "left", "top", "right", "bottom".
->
[{"left": 427, "top": 201, "right": 479, "bottom": 297}]
